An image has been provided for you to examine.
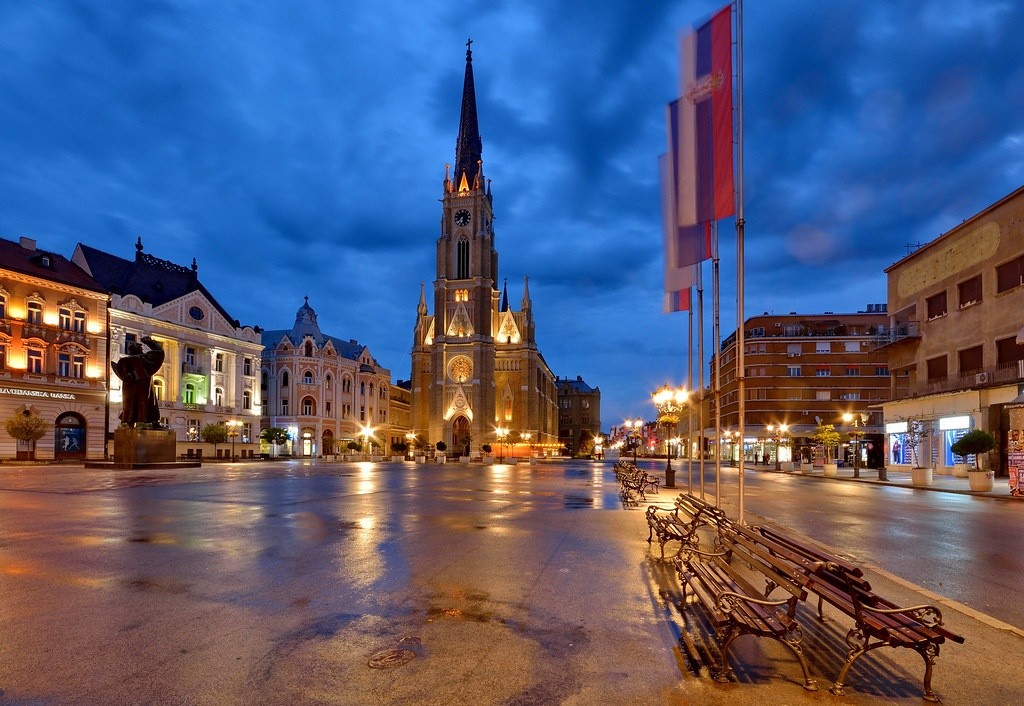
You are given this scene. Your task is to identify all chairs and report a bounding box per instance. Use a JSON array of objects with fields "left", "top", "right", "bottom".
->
[
  {"left": 645, "top": 493, "right": 707, "bottom": 563},
  {"left": 645, "top": 471, "right": 659, "bottom": 492},
  {"left": 750, "top": 523, "right": 965, "bottom": 703},
  {"left": 682, "top": 493, "right": 745, "bottom": 567},
  {"left": 670, "top": 521, "right": 826, "bottom": 693},
  {"left": 612, "top": 458, "right": 644, "bottom": 508},
  {"left": 177, "top": 448, "right": 270, "bottom": 461}
]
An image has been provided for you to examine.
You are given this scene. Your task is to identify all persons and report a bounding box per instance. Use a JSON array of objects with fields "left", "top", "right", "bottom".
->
[
  {"left": 1010, "top": 429, "right": 1020, "bottom": 442},
  {"left": 893, "top": 440, "right": 901, "bottom": 464},
  {"left": 754, "top": 451, "right": 771, "bottom": 466},
  {"left": 110, "top": 333, "right": 166, "bottom": 432}
]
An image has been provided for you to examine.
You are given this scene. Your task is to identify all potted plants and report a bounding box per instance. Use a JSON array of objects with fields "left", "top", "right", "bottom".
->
[
  {"left": 354, "top": 445, "right": 362, "bottom": 461},
  {"left": 773, "top": 428, "right": 795, "bottom": 472},
  {"left": 436, "top": 441, "right": 447, "bottom": 464},
  {"left": 528, "top": 435, "right": 539, "bottom": 465},
  {"left": 808, "top": 418, "right": 841, "bottom": 477},
  {"left": 412, "top": 434, "right": 428, "bottom": 464},
  {"left": 482, "top": 444, "right": 493, "bottom": 465},
  {"left": 398, "top": 443, "right": 408, "bottom": 463},
  {"left": 390, "top": 442, "right": 400, "bottom": 463},
  {"left": 345, "top": 442, "right": 357, "bottom": 462},
  {"left": 322, "top": 435, "right": 335, "bottom": 463},
  {"left": 458, "top": 436, "right": 472, "bottom": 464},
  {"left": 949, "top": 428, "right": 995, "bottom": 493},
  {"left": 797, "top": 447, "right": 814, "bottom": 474},
  {"left": 505, "top": 431, "right": 521, "bottom": 466},
  {"left": 335, "top": 440, "right": 344, "bottom": 462},
  {"left": 893, "top": 412, "right": 933, "bottom": 487}
]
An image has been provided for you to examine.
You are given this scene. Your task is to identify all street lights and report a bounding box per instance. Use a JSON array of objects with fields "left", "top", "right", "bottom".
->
[
  {"left": 724, "top": 430, "right": 740, "bottom": 466},
  {"left": 766, "top": 423, "right": 788, "bottom": 471},
  {"left": 225, "top": 420, "right": 243, "bottom": 463},
  {"left": 626, "top": 417, "right": 644, "bottom": 468},
  {"left": 842, "top": 413, "right": 860, "bottom": 478},
  {"left": 493, "top": 424, "right": 511, "bottom": 464},
  {"left": 650, "top": 382, "right": 689, "bottom": 489}
]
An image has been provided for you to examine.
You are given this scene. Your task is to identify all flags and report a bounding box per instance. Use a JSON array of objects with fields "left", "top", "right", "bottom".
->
[
  {"left": 665, "top": 95, "right": 721, "bottom": 270},
  {"left": 655, "top": 148, "right": 693, "bottom": 315},
  {"left": 675, "top": 3, "right": 735, "bottom": 228}
]
